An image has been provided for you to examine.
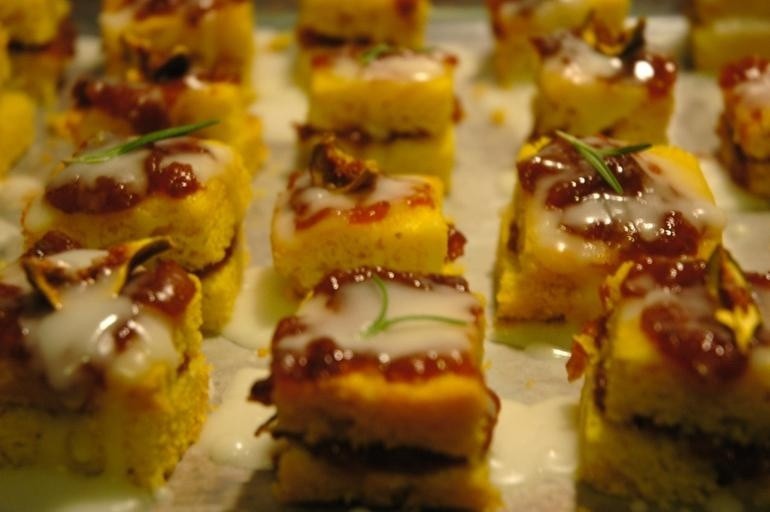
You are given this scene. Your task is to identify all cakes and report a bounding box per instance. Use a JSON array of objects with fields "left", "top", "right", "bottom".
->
[
  {"left": 0, "top": 0, "right": 76, "bottom": 97},
  {"left": 491, "top": 132, "right": 719, "bottom": 329},
  {"left": 532, "top": 29, "right": 675, "bottom": 144},
  {"left": 270, "top": 163, "right": 463, "bottom": 296},
  {"left": 254, "top": 265, "right": 501, "bottom": 511},
  {"left": 20, "top": 132, "right": 252, "bottom": 338},
  {"left": 1, "top": 246, "right": 215, "bottom": 488},
  {"left": 0, "top": 94, "right": 37, "bottom": 172},
  {"left": 715, "top": 56, "right": 770, "bottom": 200},
  {"left": 95, "top": 0, "right": 256, "bottom": 95},
  {"left": 573, "top": 255, "right": 770, "bottom": 512},
  {"left": 293, "top": 0, "right": 428, "bottom": 64},
  {"left": 691, "top": 2, "right": 770, "bottom": 66},
  {"left": 490, "top": 0, "right": 631, "bottom": 81},
  {"left": 64, "top": 77, "right": 237, "bottom": 142},
  {"left": 296, "top": 45, "right": 458, "bottom": 197}
]
